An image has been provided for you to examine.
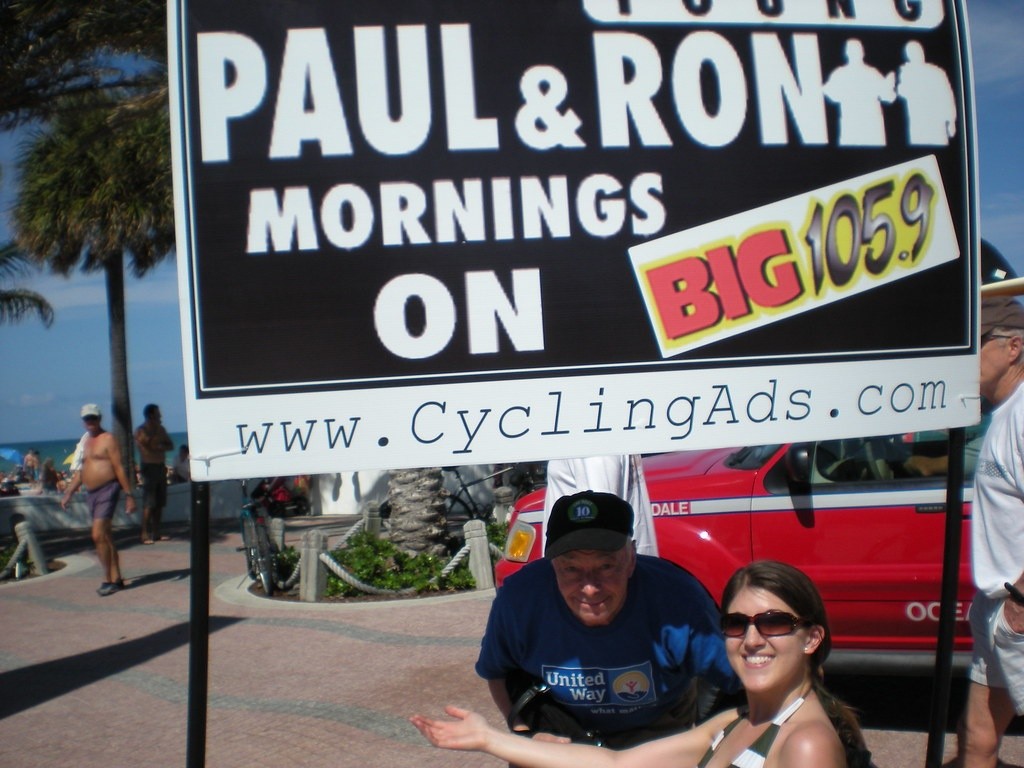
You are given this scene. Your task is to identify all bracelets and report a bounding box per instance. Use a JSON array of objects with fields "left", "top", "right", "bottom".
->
[
  {"left": 125, "top": 492, "right": 133, "bottom": 497},
  {"left": 1005, "top": 582, "right": 1023, "bottom": 608}
]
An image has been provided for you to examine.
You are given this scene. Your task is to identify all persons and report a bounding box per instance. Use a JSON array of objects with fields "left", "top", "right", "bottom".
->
[
  {"left": 134, "top": 404, "right": 174, "bottom": 546},
  {"left": 409, "top": 560, "right": 871, "bottom": 768},
  {"left": 899, "top": 411, "right": 992, "bottom": 478},
  {"left": 953, "top": 293, "right": 1023, "bottom": 768},
  {"left": 0, "top": 447, "right": 86, "bottom": 499},
  {"left": 61, "top": 402, "right": 138, "bottom": 597},
  {"left": 170, "top": 443, "right": 189, "bottom": 485},
  {"left": 474, "top": 490, "right": 748, "bottom": 768}
]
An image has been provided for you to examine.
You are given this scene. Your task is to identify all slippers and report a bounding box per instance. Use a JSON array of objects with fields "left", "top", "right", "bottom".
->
[
  {"left": 151, "top": 534, "right": 170, "bottom": 541},
  {"left": 139, "top": 537, "right": 153, "bottom": 545}
]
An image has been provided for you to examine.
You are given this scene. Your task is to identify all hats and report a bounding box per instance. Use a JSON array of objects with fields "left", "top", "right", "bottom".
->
[
  {"left": 980, "top": 295, "right": 1024, "bottom": 337},
  {"left": 544, "top": 489, "right": 634, "bottom": 559},
  {"left": 81, "top": 404, "right": 100, "bottom": 417}
]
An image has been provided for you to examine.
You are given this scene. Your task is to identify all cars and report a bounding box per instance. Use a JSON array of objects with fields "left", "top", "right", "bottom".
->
[{"left": 494, "top": 391, "right": 993, "bottom": 704}]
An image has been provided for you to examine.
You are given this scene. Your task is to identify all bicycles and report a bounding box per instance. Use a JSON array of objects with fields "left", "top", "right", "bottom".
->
[
  {"left": 236, "top": 479, "right": 281, "bottom": 595},
  {"left": 445, "top": 462, "right": 548, "bottom": 520}
]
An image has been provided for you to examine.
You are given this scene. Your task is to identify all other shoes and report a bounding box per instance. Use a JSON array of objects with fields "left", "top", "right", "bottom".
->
[{"left": 96, "top": 579, "right": 125, "bottom": 595}]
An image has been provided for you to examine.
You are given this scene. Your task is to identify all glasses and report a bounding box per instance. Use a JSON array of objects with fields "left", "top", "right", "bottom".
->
[
  {"left": 83, "top": 416, "right": 98, "bottom": 421},
  {"left": 721, "top": 611, "right": 813, "bottom": 637}
]
